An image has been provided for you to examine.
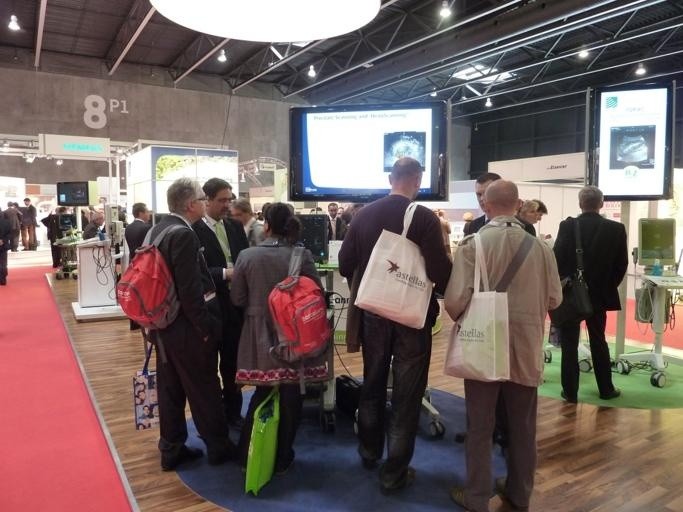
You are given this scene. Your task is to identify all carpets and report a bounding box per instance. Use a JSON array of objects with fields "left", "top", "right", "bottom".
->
[
  {"left": 175, "top": 376, "right": 509, "bottom": 512},
  {"left": 537, "top": 335, "right": 683, "bottom": 408}
]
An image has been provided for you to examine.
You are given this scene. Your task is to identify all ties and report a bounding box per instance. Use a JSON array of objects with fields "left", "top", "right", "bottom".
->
[{"left": 215, "top": 223, "right": 233, "bottom": 290}]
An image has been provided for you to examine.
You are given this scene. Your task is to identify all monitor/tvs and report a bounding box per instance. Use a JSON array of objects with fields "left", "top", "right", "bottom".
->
[
  {"left": 295, "top": 214, "right": 329, "bottom": 264},
  {"left": 104, "top": 203, "right": 118, "bottom": 223},
  {"left": 638, "top": 218, "right": 676, "bottom": 264},
  {"left": 57, "top": 182, "right": 89, "bottom": 206},
  {"left": 589, "top": 82, "right": 673, "bottom": 200},
  {"left": 289, "top": 100, "right": 451, "bottom": 202}
]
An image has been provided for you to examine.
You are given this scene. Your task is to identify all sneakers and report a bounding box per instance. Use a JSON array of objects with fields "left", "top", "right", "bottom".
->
[
  {"left": 380, "top": 468, "right": 414, "bottom": 495},
  {"left": 496, "top": 477, "right": 527, "bottom": 512},
  {"left": 450, "top": 486, "right": 465, "bottom": 507}
]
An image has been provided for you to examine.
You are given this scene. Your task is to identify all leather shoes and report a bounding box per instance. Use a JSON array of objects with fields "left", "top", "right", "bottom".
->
[
  {"left": 162, "top": 447, "right": 202, "bottom": 472},
  {"left": 560, "top": 390, "right": 576, "bottom": 403},
  {"left": 600, "top": 387, "right": 620, "bottom": 400}
]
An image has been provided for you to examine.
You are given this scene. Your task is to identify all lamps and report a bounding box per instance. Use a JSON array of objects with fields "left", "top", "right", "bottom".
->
[
  {"left": 8, "top": 15, "right": 20, "bottom": 31},
  {"left": 578, "top": 44, "right": 589, "bottom": 58},
  {"left": 307, "top": 65, "right": 316, "bottom": 77},
  {"left": 217, "top": 50, "right": 227, "bottom": 62},
  {"left": 431, "top": 87, "right": 437, "bottom": 96},
  {"left": 440, "top": 0, "right": 452, "bottom": 17},
  {"left": 485, "top": 98, "right": 493, "bottom": 107},
  {"left": 635, "top": 63, "right": 647, "bottom": 75}
]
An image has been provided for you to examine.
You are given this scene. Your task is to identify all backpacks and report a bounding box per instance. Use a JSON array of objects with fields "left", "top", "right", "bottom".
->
[{"left": 116, "top": 225, "right": 189, "bottom": 329}]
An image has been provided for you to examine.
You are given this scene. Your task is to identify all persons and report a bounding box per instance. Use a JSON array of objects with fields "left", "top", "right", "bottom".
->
[
  {"left": 125, "top": 158, "right": 629, "bottom": 511},
  {"left": 1, "top": 196, "right": 127, "bottom": 285}
]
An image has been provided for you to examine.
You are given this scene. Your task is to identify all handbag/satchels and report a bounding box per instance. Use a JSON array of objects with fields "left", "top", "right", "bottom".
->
[
  {"left": 443, "top": 292, "right": 510, "bottom": 383},
  {"left": 547, "top": 276, "right": 593, "bottom": 330},
  {"left": 354, "top": 230, "right": 432, "bottom": 330},
  {"left": 245, "top": 407, "right": 279, "bottom": 496},
  {"left": 263, "top": 276, "right": 330, "bottom": 363},
  {"left": 133, "top": 370, "right": 160, "bottom": 430}
]
